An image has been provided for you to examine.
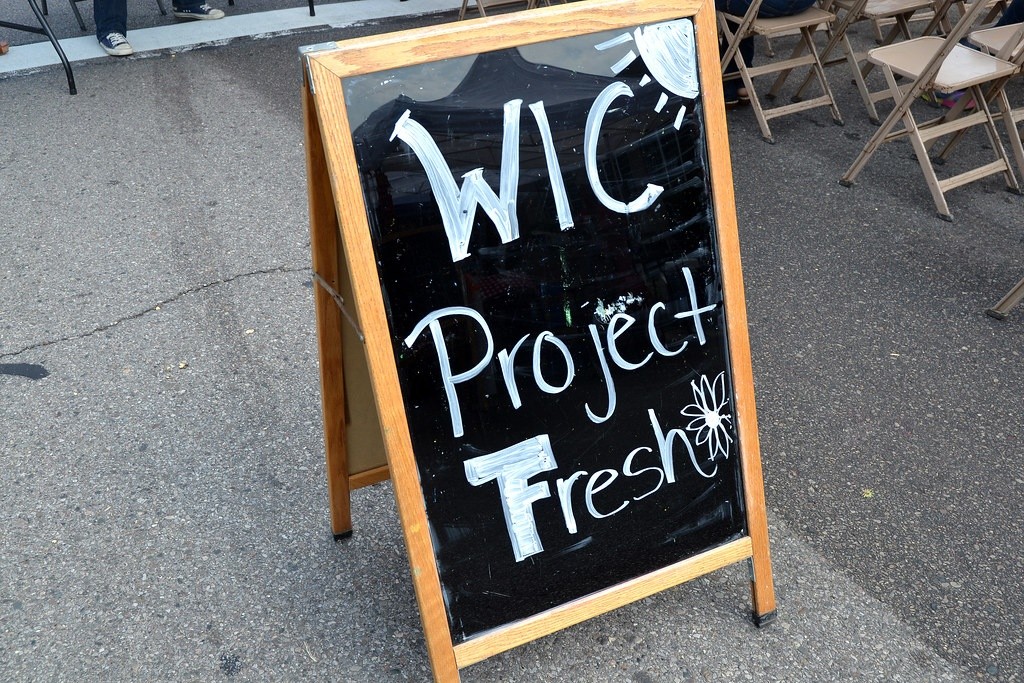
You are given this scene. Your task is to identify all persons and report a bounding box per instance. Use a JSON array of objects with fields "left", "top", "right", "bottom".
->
[
  {"left": 95, "top": 1, "right": 230, "bottom": 55},
  {"left": 714, "top": 0, "right": 819, "bottom": 106}
]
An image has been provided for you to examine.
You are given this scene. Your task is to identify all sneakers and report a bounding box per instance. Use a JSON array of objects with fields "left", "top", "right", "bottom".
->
[
  {"left": 99, "top": 33, "right": 132, "bottom": 56},
  {"left": 174, "top": 4, "right": 224, "bottom": 19}
]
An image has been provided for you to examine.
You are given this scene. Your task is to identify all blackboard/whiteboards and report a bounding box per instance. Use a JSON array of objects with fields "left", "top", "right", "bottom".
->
[{"left": 311, "top": 0, "right": 762, "bottom": 673}]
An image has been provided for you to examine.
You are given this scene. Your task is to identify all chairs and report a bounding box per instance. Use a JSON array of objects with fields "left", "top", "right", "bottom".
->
[{"left": 720, "top": 0, "right": 1023, "bottom": 320}]
